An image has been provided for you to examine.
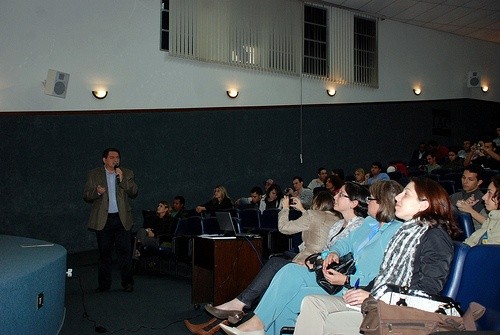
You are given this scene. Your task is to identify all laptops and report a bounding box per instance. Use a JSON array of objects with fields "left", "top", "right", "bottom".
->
[{"left": 216, "top": 212, "right": 259, "bottom": 238}]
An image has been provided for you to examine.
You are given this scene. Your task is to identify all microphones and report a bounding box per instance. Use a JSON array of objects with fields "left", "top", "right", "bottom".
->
[{"left": 114, "top": 165, "right": 120, "bottom": 184}]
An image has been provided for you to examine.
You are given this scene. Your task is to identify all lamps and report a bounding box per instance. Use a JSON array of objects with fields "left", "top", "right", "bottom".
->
[
  {"left": 413, "top": 89, "right": 422, "bottom": 95},
  {"left": 226, "top": 91, "right": 241, "bottom": 98},
  {"left": 327, "top": 89, "right": 337, "bottom": 97},
  {"left": 481, "top": 87, "right": 490, "bottom": 92},
  {"left": 92, "top": 90, "right": 108, "bottom": 99}
]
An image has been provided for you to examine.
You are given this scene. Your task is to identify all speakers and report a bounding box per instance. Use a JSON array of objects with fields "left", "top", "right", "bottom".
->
[
  {"left": 44, "top": 68, "right": 70, "bottom": 98},
  {"left": 467, "top": 71, "right": 481, "bottom": 88}
]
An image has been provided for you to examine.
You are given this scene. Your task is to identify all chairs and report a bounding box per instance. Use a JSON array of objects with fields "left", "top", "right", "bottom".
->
[
  {"left": 368, "top": 168, "right": 500, "bottom": 335},
  {"left": 128, "top": 211, "right": 300, "bottom": 281}
]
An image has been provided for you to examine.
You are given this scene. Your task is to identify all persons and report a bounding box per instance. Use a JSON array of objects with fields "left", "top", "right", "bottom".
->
[
  {"left": 135, "top": 126, "right": 500, "bottom": 335},
  {"left": 82, "top": 148, "right": 137, "bottom": 293}
]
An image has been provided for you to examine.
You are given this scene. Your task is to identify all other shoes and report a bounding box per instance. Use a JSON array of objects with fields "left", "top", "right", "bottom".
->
[
  {"left": 219, "top": 323, "right": 265, "bottom": 335},
  {"left": 123, "top": 284, "right": 133, "bottom": 292},
  {"left": 95, "top": 286, "right": 112, "bottom": 292}
]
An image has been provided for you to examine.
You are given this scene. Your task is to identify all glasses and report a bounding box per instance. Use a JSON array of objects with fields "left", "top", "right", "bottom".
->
[
  {"left": 365, "top": 197, "right": 378, "bottom": 204},
  {"left": 336, "top": 192, "right": 350, "bottom": 198}
]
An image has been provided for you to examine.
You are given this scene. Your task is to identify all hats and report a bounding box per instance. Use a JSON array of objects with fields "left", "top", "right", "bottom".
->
[{"left": 266, "top": 179, "right": 273, "bottom": 184}]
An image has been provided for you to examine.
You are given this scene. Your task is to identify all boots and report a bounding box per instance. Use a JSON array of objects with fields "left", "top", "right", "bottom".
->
[
  {"left": 135, "top": 243, "right": 143, "bottom": 261},
  {"left": 184, "top": 317, "right": 230, "bottom": 335}
]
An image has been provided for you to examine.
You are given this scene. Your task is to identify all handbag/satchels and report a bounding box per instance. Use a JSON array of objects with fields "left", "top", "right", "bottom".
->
[
  {"left": 315, "top": 252, "right": 356, "bottom": 295},
  {"left": 360, "top": 295, "right": 485, "bottom": 335},
  {"left": 305, "top": 253, "right": 323, "bottom": 272}
]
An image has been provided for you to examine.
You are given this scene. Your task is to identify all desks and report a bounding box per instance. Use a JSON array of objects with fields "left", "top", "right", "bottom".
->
[{"left": 192, "top": 236, "right": 263, "bottom": 306}]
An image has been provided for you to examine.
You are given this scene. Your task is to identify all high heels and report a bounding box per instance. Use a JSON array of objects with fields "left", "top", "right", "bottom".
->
[{"left": 205, "top": 305, "right": 244, "bottom": 324}]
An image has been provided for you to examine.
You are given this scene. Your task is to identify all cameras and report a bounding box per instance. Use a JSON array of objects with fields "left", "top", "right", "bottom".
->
[
  {"left": 289, "top": 199, "right": 294, "bottom": 205},
  {"left": 476, "top": 143, "right": 481, "bottom": 150},
  {"left": 285, "top": 189, "right": 290, "bottom": 192}
]
